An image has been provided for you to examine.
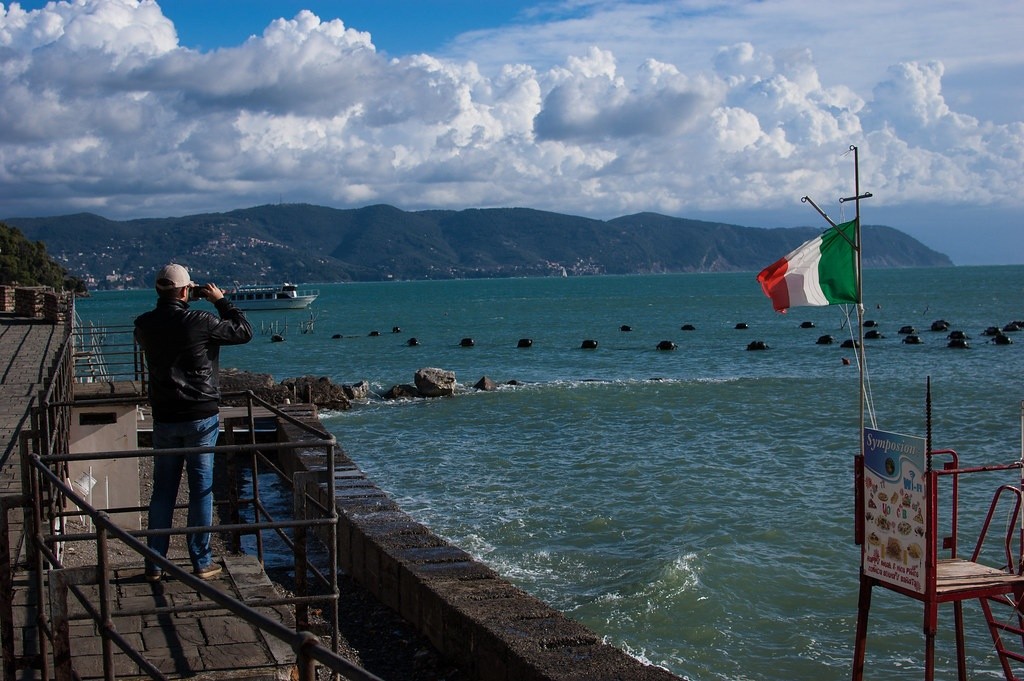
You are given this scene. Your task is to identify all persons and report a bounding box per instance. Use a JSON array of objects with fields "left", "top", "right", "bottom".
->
[{"left": 133, "top": 264, "right": 252, "bottom": 579}]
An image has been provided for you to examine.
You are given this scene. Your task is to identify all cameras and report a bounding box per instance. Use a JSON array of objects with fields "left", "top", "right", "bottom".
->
[{"left": 193, "top": 286, "right": 210, "bottom": 297}]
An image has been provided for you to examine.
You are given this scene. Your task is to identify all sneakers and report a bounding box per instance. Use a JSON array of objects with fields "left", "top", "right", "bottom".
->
[
  {"left": 193, "top": 560, "right": 223, "bottom": 580},
  {"left": 145, "top": 569, "right": 166, "bottom": 583}
]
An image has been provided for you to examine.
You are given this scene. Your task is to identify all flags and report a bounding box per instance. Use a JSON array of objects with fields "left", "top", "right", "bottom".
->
[{"left": 756, "top": 218, "right": 858, "bottom": 315}]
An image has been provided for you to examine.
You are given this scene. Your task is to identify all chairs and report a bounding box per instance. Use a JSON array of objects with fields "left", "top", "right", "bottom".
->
[{"left": 852, "top": 447, "right": 1024, "bottom": 681}]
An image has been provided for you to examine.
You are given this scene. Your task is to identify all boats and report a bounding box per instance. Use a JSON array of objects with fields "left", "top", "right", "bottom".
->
[{"left": 222, "top": 281, "right": 318, "bottom": 310}]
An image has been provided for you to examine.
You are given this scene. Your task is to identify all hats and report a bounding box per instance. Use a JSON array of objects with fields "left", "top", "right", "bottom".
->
[{"left": 156, "top": 263, "right": 200, "bottom": 290}]
